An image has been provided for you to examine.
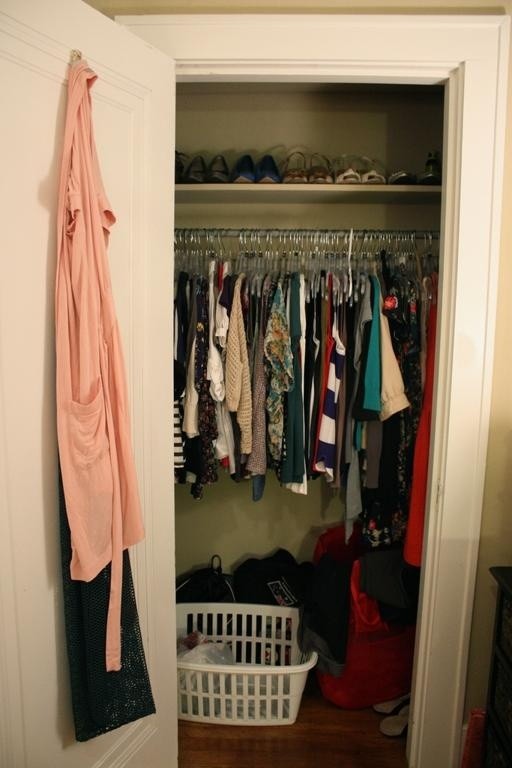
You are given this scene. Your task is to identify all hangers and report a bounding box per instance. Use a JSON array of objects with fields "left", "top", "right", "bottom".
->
[{"left": 173, "top": 224, "right": 441, "bottom": 310}]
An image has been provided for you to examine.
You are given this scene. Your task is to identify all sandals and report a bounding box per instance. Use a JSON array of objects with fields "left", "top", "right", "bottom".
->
[{"left": 281, "top": 151, "right": 441, "bottom": 185}]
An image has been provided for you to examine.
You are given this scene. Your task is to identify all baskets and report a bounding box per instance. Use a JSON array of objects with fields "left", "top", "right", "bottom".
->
[{"left": 176, "top": 602, "right": 319, "bottom": 727}]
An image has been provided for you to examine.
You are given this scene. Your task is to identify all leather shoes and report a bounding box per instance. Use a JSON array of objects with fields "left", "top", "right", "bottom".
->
[{"left": 175, "top": 150, "right": 281, "bottom": 184}]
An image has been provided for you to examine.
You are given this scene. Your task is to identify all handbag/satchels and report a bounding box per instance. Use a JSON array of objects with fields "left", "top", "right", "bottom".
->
[
  {"left": 176, "top": 554, "right": 243, "bottom": 602},
  {"left": 312, "top": 523, "right": 416, "bottom": 711}
]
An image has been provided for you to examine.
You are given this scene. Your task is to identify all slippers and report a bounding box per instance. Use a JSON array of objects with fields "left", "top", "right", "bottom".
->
[{"left": 373, "top": 694, "right": 411, "bottom": 736}]
